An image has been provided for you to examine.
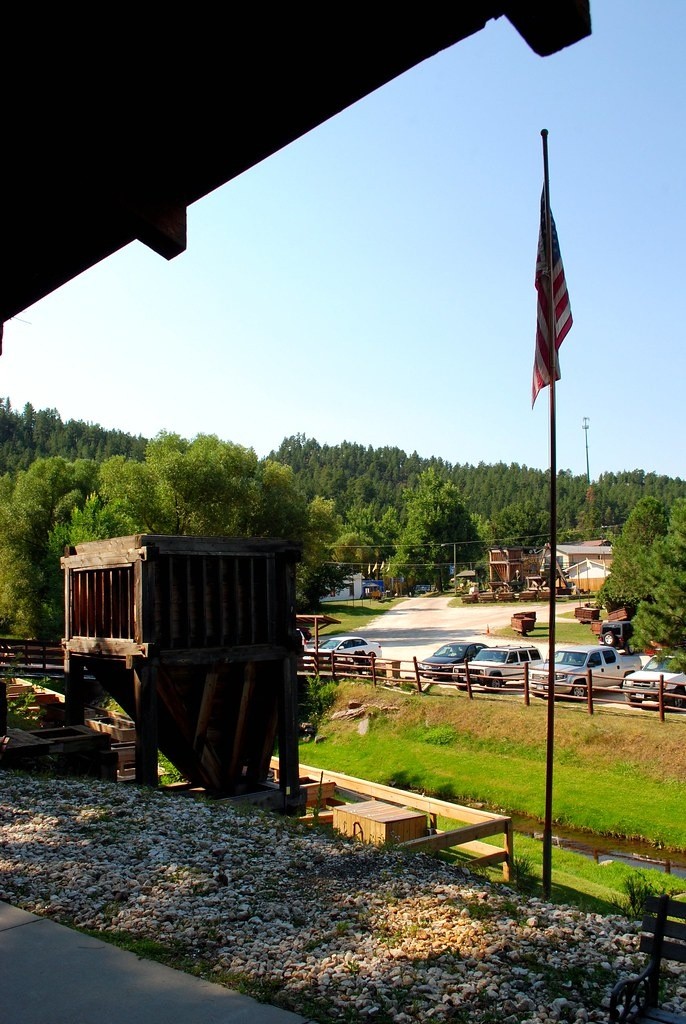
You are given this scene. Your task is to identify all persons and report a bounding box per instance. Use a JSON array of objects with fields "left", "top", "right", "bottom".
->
[{"left": 513, "top": 569, "right": 521, "bottom": 582}]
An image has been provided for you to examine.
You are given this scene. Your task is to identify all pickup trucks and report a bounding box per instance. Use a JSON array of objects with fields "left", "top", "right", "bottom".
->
[{"left": 529, "top": 644, "right": 641, "bottom": 702}]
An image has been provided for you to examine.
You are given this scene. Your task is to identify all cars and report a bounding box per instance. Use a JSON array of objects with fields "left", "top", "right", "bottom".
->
[
  {"left": 303, "top": 637, "right": 382, "bottom": 667},
  {"left": 417, "top": 642, "right": 490, "bottom": 681}
]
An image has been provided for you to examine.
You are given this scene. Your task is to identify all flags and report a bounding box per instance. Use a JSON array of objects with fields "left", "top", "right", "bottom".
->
[{"left": 532, "top": 181, "right": 574, "bottom": 410}]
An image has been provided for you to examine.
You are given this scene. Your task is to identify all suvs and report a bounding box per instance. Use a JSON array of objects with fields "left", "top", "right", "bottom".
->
[
  {"left": 452, "top": 644, "right": 543, "bottom": 693},
  {"left": 644, "top": 639, "right": 686, "bottom": 657},
  {"left": 599, "top": 621, "right": 642, "bottom": 654},
  {"left": 622, "top": 651, "right": 686, "bottom": 713},
  {"left": 297, "top": 627, "right": 307, "bottom": 649}
]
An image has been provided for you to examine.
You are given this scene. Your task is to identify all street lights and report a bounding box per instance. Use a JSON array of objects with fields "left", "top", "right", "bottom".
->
[{"left": 441, "top": 543, "right": 457, "bottom": 596}]
